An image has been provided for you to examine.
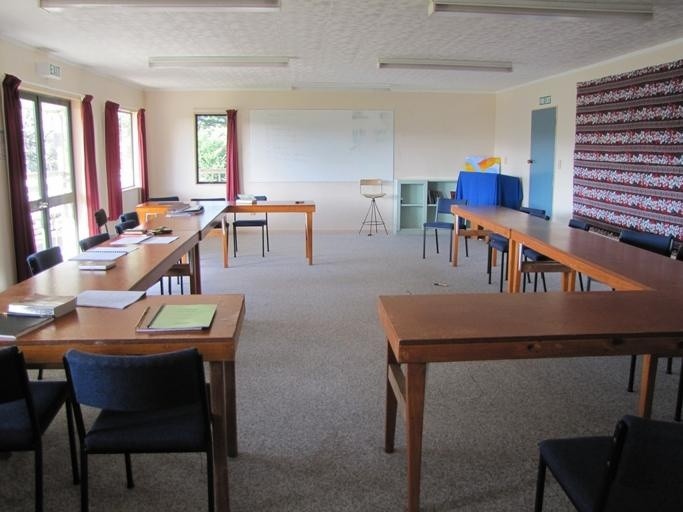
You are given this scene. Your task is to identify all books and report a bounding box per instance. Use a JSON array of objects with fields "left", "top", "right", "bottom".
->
[
  {"left": 166, "top": 204, "right": 204, "bottom": 217},
  {"left": 69, "top": 225, "right": 178, "bottom": 270},
  {"left": 0, "top": 294, "right": 78, "bottom": 340},
  {"left": 235, "top": 194, "right": 257, "bottom": 204},
  {"left": 135, "top": 303, "right": 217, "bottom": 333}
]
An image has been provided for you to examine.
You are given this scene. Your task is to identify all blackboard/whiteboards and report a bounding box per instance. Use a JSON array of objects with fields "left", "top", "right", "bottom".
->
[{"left": 247, "top": 106, "right": 394, "bottom": 184}]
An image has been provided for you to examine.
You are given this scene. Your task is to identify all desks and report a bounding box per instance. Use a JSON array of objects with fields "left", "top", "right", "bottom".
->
[
  {"left": 1, "top": 293, "right": 246, "bottom": 512},
  {"left": 450, "top": 204, "right": 555, "bottom": 293},
  {"left": 126, "top": 200, "right": 317, "bottom": 266},
  {"left": 378, "top": 289, "right": 683, "bottom": 511},
  {"left": 1, "top": 231, "right": 201, "bottom": 293},
  {"left": 511, "top": 222, "right": 683, "bottom": 289}
]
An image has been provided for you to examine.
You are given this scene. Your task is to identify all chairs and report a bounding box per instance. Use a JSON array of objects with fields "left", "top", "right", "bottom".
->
[
  {"left": 533, "top": 414, "right": 683, "bottom": 510},
  {"left": 190, "top": 198, "right": 229, "bottom": 255},
  {"left": 26, "top": 246, "right": 63, "bottom": 277},
  {"left": 114, "top": 211, "right": 140, "bottom": 235},
  {"left": 80, "top": 232, "right": 110, "bottom": 253},
  {"left": 232, "top": 195, "right": 270, "bottom": 258},
  {"left": 61, "top": 347, "right": 216, "bottom": 511},
  {"left": 94, "top": 209, "right": 109, "bottom": 234},
  {"left": 358, "top": 179, "right": 389, "bottom": 236},
  {"left": 522, "top": 219, "right": 590, "bottom": 293},
  {"left": 1, "top": 345, "right": 79, "bottom": 511},
  {"left": 422, "top": 197, "right": 470, "bottom": 262},
  {"left": 619, "top": 228, "right": 674, "bottom": 257},
  {"left": 486, "top": 206, "right": 550, "bottom": 293},
  {"left": 675, "top": 246, "right": 683, "bottom": 261},
  {"left": 148, "top": 196, "right": 179, "bottom": 201}
]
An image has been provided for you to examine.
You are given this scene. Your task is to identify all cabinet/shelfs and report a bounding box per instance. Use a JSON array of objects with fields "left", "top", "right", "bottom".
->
[{"left": 394, "top": 179, "right": 460, "bottom": 236}]
